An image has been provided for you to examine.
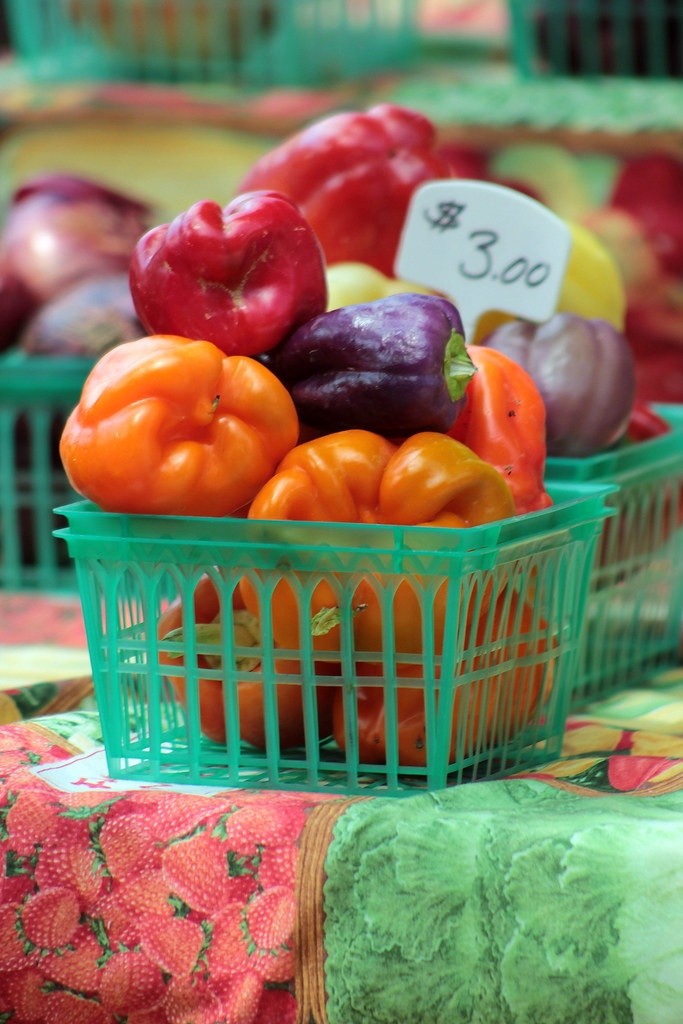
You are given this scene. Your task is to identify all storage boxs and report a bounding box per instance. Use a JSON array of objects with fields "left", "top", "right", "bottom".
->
[
  {"left": 5, "top": 0, "right": 421, "bottom": 92},
  {"left": 52, "top": 482, "right": 619, "bottom": 796},
  {"left": 545, "top": 400, "right": 682, "bottom": 708},
  {"left": 504, "top": 3, "right": 679, "bottom": 95},
  {"left": 0, "top": 350, "right": 100, "bottom": 592}
]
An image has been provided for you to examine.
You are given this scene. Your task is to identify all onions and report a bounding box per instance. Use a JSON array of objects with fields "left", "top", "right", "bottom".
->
[{"left": 0, "top": 174, "right": 160, "bottom": 564}]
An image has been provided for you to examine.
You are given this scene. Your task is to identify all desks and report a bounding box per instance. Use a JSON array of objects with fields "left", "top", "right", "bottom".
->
[{"left": 0, "top": 581, "right": 678, "bottom": 1021}]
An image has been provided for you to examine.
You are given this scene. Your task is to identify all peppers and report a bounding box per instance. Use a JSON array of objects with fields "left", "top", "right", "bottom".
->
[{"left": 57, "top": 101, "right": 683, "bottom": 773}]
